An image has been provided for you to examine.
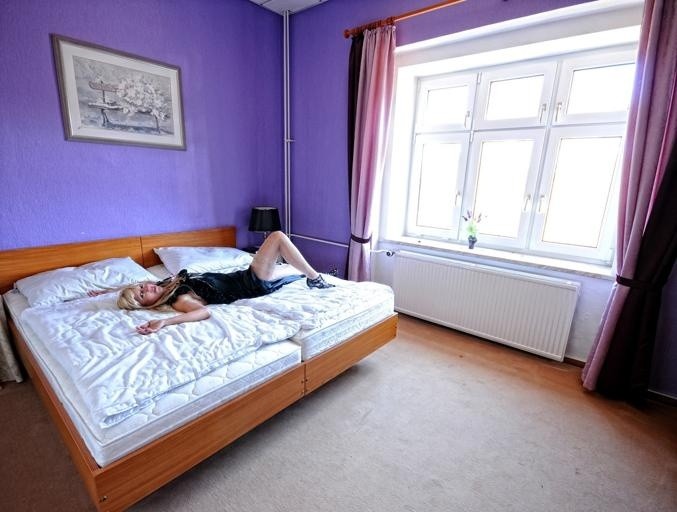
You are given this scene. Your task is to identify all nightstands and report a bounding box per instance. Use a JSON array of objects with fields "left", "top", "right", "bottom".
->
[{"left": 239, "top": 243, "right": 262, "bottom": 255}]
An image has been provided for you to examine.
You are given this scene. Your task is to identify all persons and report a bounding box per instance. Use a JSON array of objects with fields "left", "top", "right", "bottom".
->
[{"left": 88, "top": 231, "right": 338, "bottom": 335}]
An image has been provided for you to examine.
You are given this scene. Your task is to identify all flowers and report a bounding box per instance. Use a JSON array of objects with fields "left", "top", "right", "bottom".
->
[{"left": 460, "top": 208, "right": 484, "bottom": 239}]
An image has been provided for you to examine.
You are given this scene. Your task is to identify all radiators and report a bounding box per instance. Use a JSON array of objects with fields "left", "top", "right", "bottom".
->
[{"left": 391, "top": 246, "right": 582, "bottom": 364}]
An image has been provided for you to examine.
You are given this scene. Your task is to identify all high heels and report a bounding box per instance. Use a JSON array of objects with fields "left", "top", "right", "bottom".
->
[{"left": 306, "top": 273, "right": 336, "bottom": 290}]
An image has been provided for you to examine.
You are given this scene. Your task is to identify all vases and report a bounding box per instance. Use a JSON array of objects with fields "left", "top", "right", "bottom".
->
[{"left": 467, "top": 234, "right": 477, "bottom": 249}]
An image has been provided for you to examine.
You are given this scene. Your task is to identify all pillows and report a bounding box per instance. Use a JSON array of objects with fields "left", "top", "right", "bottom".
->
[
  {"left": 12, "top": 256, "right": 159, "bottom": 310},
  {"left": 151, "top": 245, "right": 254, "bottom": 280}
]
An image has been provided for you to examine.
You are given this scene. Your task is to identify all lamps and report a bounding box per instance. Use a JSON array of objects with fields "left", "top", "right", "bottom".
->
[{"left": 247, "top": 205, "right": 282, "bottom": 241}]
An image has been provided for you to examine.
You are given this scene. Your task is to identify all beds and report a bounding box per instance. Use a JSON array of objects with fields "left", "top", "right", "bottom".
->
[{"left": 0, "top": 225, "right": 397, "bottom": 512}]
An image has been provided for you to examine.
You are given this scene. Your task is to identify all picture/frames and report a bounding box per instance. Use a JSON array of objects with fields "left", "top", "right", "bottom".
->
[{"left": 50, "top": 33, "right": 187, "bottom": 153}]
